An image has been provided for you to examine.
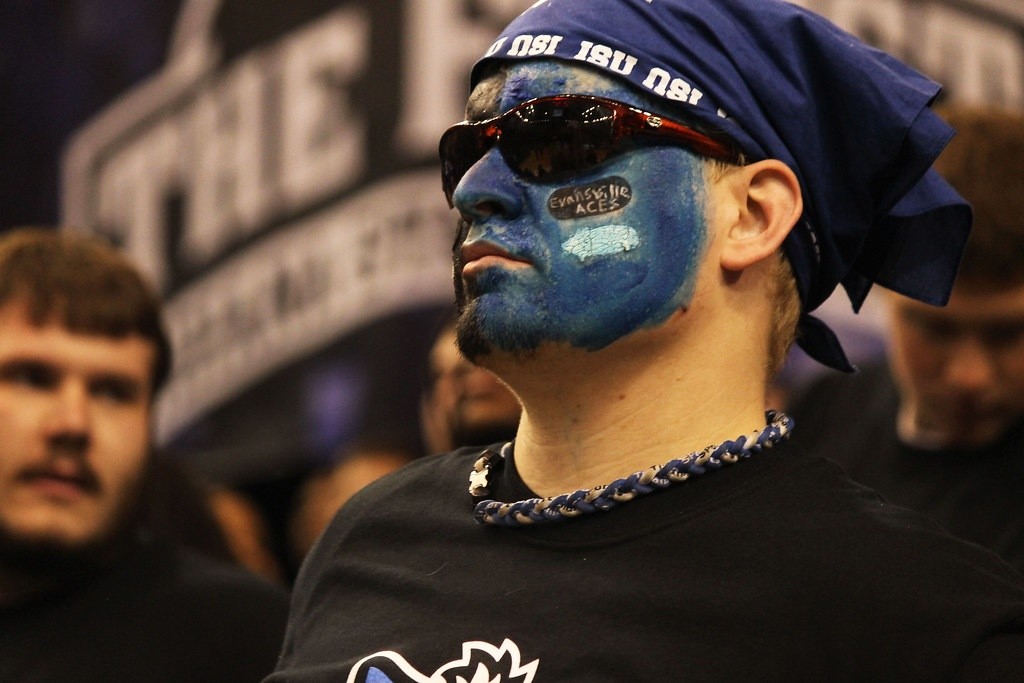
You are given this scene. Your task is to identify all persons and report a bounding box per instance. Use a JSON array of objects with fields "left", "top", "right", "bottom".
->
[
  {"left": 195, "top": 302, "right": 522, "bottom": 595},
  {"left": 0, "top": 228, "right": 290, "bottom": 683},
  {"left": 260, "top": 0, "right": 1024, "bottom": 683}
]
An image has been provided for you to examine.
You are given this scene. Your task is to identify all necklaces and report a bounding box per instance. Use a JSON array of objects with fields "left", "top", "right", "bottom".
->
[{"left": 467, "top": 410, "right": 795, "bottom": 525}]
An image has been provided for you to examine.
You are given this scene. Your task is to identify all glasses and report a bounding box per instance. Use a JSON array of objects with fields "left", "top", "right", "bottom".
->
[{"left": 439, "top": 96, "right": 754, "bottom": 210}]
